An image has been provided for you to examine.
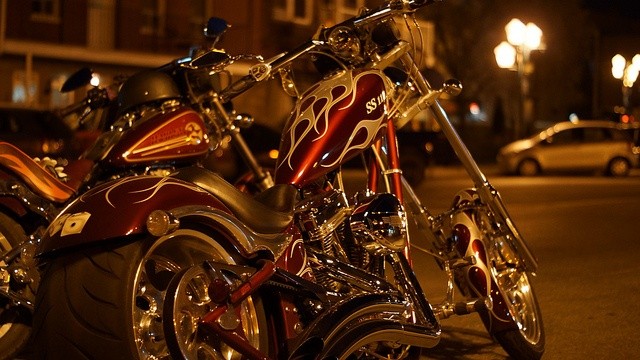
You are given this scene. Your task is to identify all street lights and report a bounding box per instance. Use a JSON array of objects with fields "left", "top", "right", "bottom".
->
[
  {"left": 611, "top": 52, "right": 640, "bottom": 108},
  {"left": 493, "top": 16, "right": 549, "bottom": 136}
]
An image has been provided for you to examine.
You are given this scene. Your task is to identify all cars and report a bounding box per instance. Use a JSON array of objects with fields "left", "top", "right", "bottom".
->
[{"left": 496, "top": 119, "right": 640, "bottom": 177}]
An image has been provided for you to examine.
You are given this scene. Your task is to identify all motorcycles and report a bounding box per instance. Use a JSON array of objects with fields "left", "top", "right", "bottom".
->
[
  {"left": 0, "top": 17, "right": 276, "bottom": 359},
  {"left": 32, "top": 1, "right": 546, "bottom": 360}
]
